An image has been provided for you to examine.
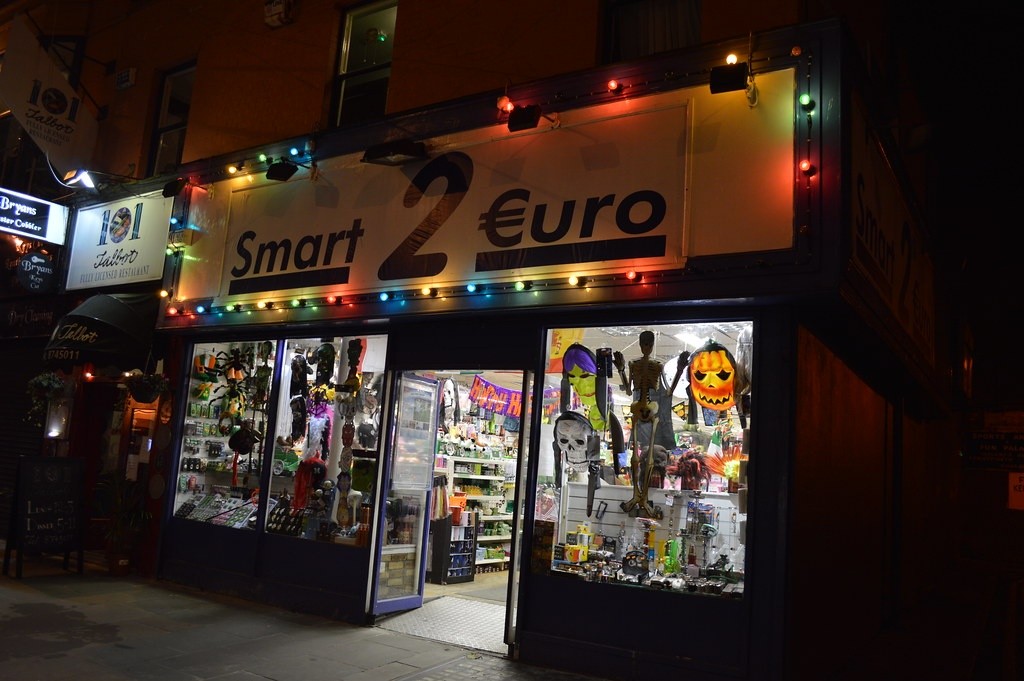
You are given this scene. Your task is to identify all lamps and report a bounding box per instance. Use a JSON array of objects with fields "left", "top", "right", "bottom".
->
[
  {"left": 508, "top": 103, "right": 560, "bottom": 131},
  {"left": 266, "top": 156, "right": 318, "bottom": 182},
  {"left": 361, "top": 139, "right": 427, "bottom": 166},
  {"left": 64, "top": 168, "right": 138, "bottom": 186},
  {"left": 162, "top": 180, "right": 215, "bottom": 199},
  {"left": 709, "top": 62, "right": 756, "bottom": 108}
]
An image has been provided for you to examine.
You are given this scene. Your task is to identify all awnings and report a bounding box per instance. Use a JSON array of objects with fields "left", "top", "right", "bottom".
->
[{"left": 45, "top": 293, "right": 164, "bottom": 369}]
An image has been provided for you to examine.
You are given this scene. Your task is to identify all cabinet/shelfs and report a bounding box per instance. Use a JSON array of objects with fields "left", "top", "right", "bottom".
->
[
  {"left": 557, "top": 452, "right": 746, "bottom": 571},
  {"left": 422, "top": 456, "right": 518, "bottom": 586}
]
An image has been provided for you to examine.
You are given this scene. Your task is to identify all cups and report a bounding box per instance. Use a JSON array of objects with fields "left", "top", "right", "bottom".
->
[
  {"left": 360, "top": 502, "right": 370, "bottom": 523},
  {"left": 449, "top": 506, "right": 462, "bottom": 525}
]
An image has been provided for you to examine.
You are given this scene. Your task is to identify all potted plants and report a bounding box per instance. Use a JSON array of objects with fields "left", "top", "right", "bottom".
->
[
  {"left": 122, "top": 373, "right": 170, "bottom": 404},
  {"left": 94, "top": 468, "right": 148, "bottom": 580},
  {"left": 21, "top": 372, "right": 66, "bottom": 430}
]
